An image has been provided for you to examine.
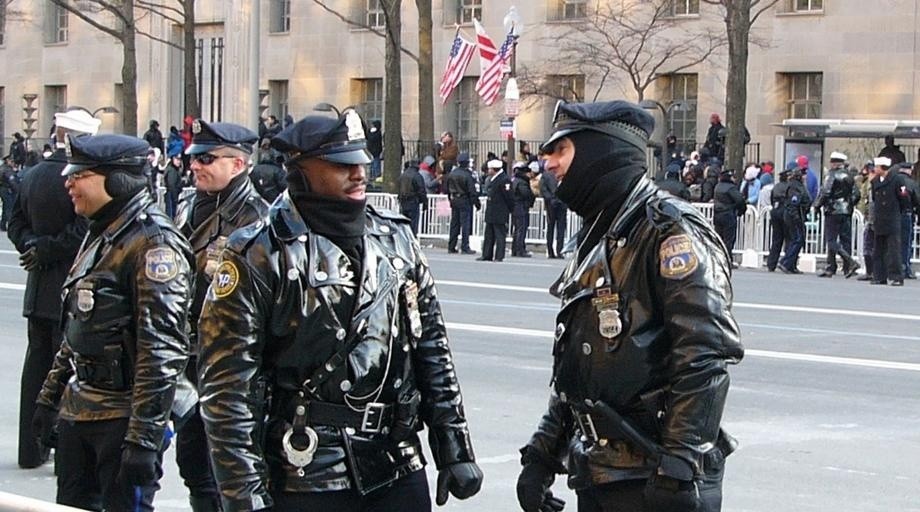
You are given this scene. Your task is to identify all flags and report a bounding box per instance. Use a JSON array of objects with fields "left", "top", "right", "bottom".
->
[
  {"left": 474, "top": 25, "right": 517, "bottom": 107},
  {"left": 439, "top": 27, "right": 473, "bottom": 103},
  {"left": 471, "top": 20, "right": 498, "bottom": 77}
]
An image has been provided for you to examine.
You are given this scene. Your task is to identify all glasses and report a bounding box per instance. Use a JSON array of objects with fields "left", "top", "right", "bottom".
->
[
  {"left": 192, "top": 153, "right": 238, "bottom": 164},
  {"left": 68, "top": 173, "right": 99, "bottom": 180}
]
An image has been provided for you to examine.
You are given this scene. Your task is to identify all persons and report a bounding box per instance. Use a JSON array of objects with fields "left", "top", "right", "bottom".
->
[
  {"left": 195, "top": 109, "right": 482, "bottom": 511},
  {"left": 1, "top": 104, "right": 300, "bottom": 512},
  {"left": 655, "top": 113, "right": 920, "bottom": 286},
  {"left": 365, "top": 120, "right": 565, "bottom": 262},
  {"left": 516, "top": 99, "right": 745, "bottom": 511}
]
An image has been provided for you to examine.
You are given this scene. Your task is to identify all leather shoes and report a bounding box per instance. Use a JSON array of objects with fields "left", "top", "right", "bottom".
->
[
  {"left": 460, "top": 249, "right": 477, "bottom": 255},
  {"left": 448, "top": 248, "right": 459, "bottom": 253},
  {"left": 476, "top": 251, "right": 565, "bottom": 262},
  {"left": 767, "top": 261, "right": 918, "bottom": 285}
]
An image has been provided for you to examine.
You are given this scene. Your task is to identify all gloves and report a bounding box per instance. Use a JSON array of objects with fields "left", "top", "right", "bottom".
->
[
  {"left": 814, "top": 207, "right": 822, "bottom": 220},
  {"left": 18, "top": 239, "right": 38, "bottom": 270},
  {"left": 642, "top": 476, "right": 701, "bottom": 512},
  {"left": 737, "top": 210, "right": 746, "bottom": 217},
  {"left": 436, "top": 463, "right": 483, "bottom": 506},
  {"left": 116, "top": 444, "right": 156, "bottom": 484},
  {"left": 423, "top": 201, "right": 428, "bottom": 211},
  {"left": 475, "top": 202, "right": 481, "bottom": 211},
  {"left": 517, "top": 464, "right": 566, "bottom": 512}
]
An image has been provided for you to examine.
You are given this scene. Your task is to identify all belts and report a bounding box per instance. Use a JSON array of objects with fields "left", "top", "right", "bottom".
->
[
  {"left": 569, "top": 409, "right": 667, "bottom": 440},
  {"left": 74, "top": 358, "right": 135, "bottom": 392},
  {"left": 270, "top": 395, "right": 395, "bottom": 432}
]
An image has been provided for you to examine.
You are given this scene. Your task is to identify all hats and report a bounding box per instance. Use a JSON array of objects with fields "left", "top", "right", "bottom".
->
[
  {"left": 541, "top": 100, "right": 655, "bottom": 155},
  {"left": 60, "top": 134, "right": 150, "bottom": 176},
  {"left": 712, "top": 114, "right": 720, "bottom": 123},
  {"left": 271, "top": 107, "right": 375, "bottom": 164},
  {"left": 407, "top": 140, "right": 542, "bottom": 178},
  {"left": 664, "top": 149, "right": 911, "bottom": 181},
  {"left": 54, "top": 109, "right": 102, "bottom": 136},
  {"left": 184, "top": 118, "right": 260, "bottom": 155}
]
